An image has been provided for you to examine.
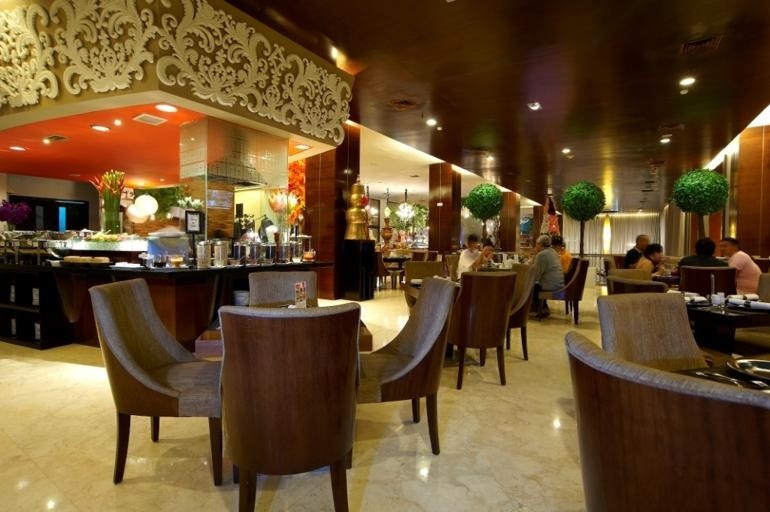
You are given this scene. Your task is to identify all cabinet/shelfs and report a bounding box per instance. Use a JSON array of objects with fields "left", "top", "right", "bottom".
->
[{"left": 0, "top": 264, "right": 58, "bottom": 351}]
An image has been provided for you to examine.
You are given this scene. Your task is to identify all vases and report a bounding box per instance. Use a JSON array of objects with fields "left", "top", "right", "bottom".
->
[
  {"left": 276, "top": 216, "right": 290, "bottom": 245},
  {"left": 103, "top": 211, "right": 120, "bottom": 234},
  {"left": 9, "top": 224, "right": 15, "bottom": 231}
]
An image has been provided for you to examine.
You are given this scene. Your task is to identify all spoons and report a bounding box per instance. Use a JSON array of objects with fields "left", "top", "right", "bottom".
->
[{"left": 706, "top": 371, "right": 769, "bottom": 389}]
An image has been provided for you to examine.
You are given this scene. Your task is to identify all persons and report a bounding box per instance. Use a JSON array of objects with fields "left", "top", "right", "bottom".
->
[
  {"left": 527, "top": 235, "right": 564, "bottom": 319},
  {"left": 483, "top": 238, "right": 494, "bottom": 265},
  {"left": 551, "top": 235, "right": 572, "bottom": 272},
  {"left": 673, "top": 237, "right": 729, "bottom": 286},
  {"left": 623, "top": 236, "right": 652, "bottom": 268},
  {"left": 717, "top": 238, "right": 761, "bottom": 293},
  {"left": 635, "top": 242, "right": 668, "bottom": 281},
  {"left": 458, "top": 235, "right": 490, "bottom": 275}
]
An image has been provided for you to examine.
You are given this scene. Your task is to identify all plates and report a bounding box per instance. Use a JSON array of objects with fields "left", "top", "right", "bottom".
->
[{"left": 726, "top": 359, "right": 770, "bottom": 381}]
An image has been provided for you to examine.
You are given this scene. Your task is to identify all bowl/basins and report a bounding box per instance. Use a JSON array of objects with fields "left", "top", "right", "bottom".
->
[{"left": 50, "top": 231, "right": 73, "bottom": 240}]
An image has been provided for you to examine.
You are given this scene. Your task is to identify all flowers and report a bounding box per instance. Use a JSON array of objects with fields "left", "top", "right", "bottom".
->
[
  {"left": 0, "top": 201, "right": 32, "bottom": 224},
  {"left": 90, "top": 171, "right": 126, "bottom": 234},
  {"left": 268, "top": 190, "right": 297, "bottom": 223}
]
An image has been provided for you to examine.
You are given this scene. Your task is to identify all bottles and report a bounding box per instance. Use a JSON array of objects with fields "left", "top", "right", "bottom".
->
[{"left": 196, "top": 241, "right": 306, "bottom": 269}]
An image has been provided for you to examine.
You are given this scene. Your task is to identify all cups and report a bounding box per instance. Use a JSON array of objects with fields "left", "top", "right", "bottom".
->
[
  {"left": 153, "top": 255, "right": 166, "bottom": 268},
  {"left": 169, "top": 255, "right": 183, "bottom": 268},
  {"left": 138, "top": 254, "right": 148, "bottom": 267}
]
